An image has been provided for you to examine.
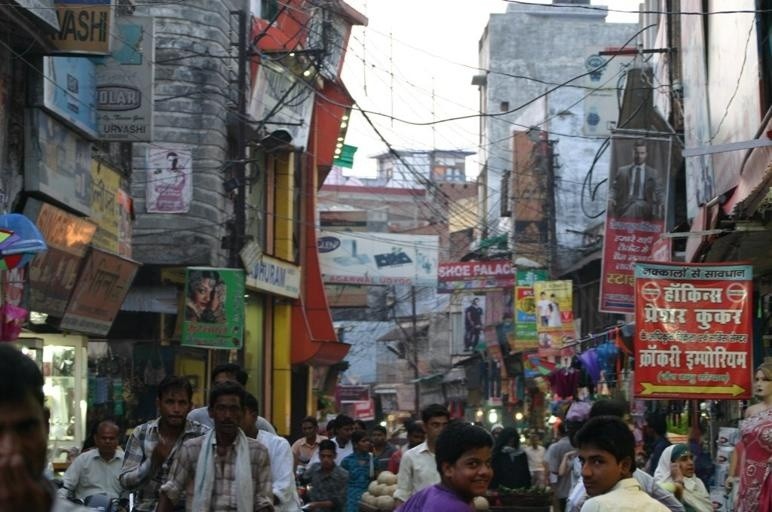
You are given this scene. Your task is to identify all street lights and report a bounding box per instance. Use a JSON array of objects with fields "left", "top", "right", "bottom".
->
[
  {"left": 226, "top": 129, "right": 293, "bottom": 266},
  {"left": 385, "top": 283, "right": 421, "bottom": 411}
]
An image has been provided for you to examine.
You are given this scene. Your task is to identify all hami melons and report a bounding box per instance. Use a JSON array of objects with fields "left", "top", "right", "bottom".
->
[
  {"left": 361, "top": 470, "right": 397, "bottom": 510},
  {"left": 472, "top": 496, "right": 489, "bottom": 510}
]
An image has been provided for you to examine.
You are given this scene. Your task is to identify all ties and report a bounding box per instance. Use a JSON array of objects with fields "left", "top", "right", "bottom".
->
[{"left": 633, "top": 167, "right": 642, "bottom": 197}]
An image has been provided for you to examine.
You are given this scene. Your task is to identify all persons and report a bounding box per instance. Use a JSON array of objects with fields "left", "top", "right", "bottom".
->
[
  {"left": 610, "top": 137, "right": 665, "bottom": 220},
  {"left": 0, "top": 341, "right": 97, "bottom": 511},
  {"left": 184, "top": 270, "right": 227, "bottom": 326},
  {"left": 546, "top": 292, "right": 560, "bottom": 326},
  {"left": 152, "top": 150, "right": 183, "bottom": 190},
  {"left": 537, "top": 290, "right": 551, "bottom": 326},
  {"left": 463, "top": 296, "right": 482, "bottom": 349}
]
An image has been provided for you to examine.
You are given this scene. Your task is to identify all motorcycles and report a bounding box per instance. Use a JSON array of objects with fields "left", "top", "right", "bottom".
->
[{"left": 55, "top": 478, "right": 132, "bottom": 512}]
